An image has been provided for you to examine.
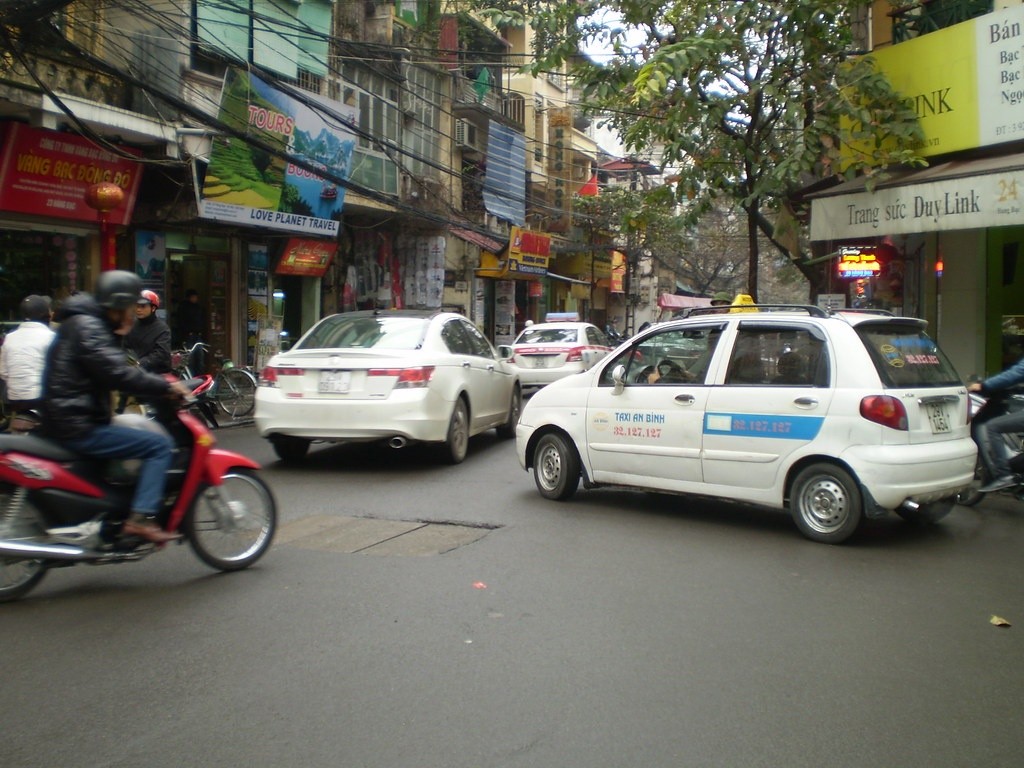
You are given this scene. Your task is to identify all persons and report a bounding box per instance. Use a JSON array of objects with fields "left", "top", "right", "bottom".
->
[
  {"left": 647, "top": 328, "right": 722, "bottom": 383},
  {"left": 967, "top": 359, "right": 1024, "bottom": 491},
  {"left": 184, "top": 289, "right": 208, "bottom": 377},
  {"left": 0, "top": 270, "right": 184, "bottom": 541}
]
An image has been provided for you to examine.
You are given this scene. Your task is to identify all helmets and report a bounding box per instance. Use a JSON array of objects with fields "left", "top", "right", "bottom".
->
[
  {"left": 136, "top": 289, "right": 159, "bottom": 306},
  {"left": 95, "top": 270, "right": 143, "bottom": 304},
  {"left": 710, "top": 292, "right": 730, "bottom": 306},
  {"left": 24, "top": 295, "right": 52, "bottom": 325}
]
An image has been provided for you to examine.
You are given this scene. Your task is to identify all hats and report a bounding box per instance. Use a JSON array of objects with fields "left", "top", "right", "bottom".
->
[{"left": 63, "top": 292, "right": 96, "bottom": 313}]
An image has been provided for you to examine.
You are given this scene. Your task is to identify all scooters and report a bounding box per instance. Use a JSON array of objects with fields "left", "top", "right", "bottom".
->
[
  {"left": 955, "top": 390, "right": 1024, "bottom": 506},
  {"left": 6, "top": 408, "right": 43, "bottom": 436}
]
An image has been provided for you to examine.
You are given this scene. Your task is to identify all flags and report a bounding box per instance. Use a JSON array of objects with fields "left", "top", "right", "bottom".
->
[{"left": 578, "top": 174, "right": 596, "bottom": 197}]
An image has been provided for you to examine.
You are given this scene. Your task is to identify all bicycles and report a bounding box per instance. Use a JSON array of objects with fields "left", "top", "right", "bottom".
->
[{"left": 171, "top": 342, "right": 256, "bottom": 420}]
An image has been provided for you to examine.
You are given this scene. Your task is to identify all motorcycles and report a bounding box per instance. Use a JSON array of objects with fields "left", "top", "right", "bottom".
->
[
  {"left": 0, "top": 373, "right": 279, "bottom": 602},
  {"left": 112, "top": 372, "right": 220, "bottom": 430}
]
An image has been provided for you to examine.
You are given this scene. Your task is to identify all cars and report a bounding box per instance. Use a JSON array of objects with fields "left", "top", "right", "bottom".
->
[
  {"left": 510, "top": 313, "right": 616, "bottom": 389},
  {"left": 514, "top": 292, "right": 979, "bottom": 545},
  {"left": 622, "top": 318, "right": 713, "bottom": 371},
  {"left": 253, "top": 309, "right": 522, "bottom": 465}
]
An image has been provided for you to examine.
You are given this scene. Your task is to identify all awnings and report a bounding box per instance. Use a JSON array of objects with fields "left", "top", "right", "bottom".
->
[{"left": 806, "top": 152, "right": 1024, "bottom": 242}]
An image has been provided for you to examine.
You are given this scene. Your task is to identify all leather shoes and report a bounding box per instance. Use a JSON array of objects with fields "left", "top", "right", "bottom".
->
[
  {"left": 124, "top": 520, "right": 182, "bottom": 542},
  {"left": 977, "top": 475, "right": 1016, "bottom": 491}
]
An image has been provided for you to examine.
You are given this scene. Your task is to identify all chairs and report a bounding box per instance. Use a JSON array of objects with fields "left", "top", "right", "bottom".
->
[{"left": 725, "top": 351, "right": 808, "bottom": 385}]
